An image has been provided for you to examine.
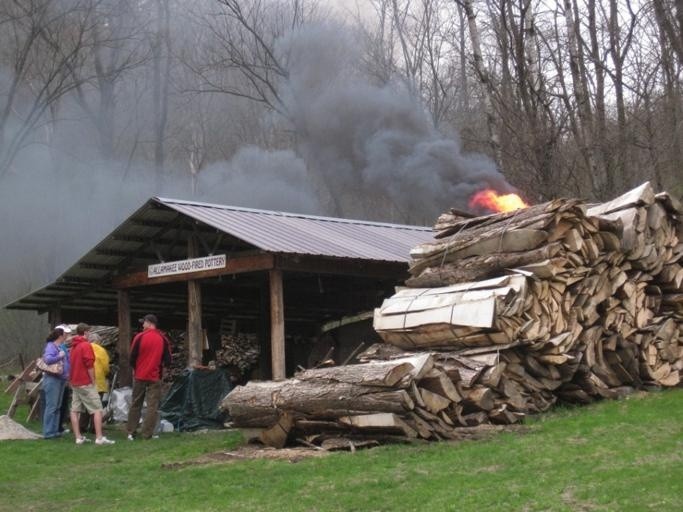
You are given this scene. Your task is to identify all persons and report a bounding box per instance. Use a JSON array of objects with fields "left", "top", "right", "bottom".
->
[
  {"left": 37, "top": 323, "right": 116, "bottom": 444},
  {"left": 128, "top": 314, "right": 172, "bottom": 443}
]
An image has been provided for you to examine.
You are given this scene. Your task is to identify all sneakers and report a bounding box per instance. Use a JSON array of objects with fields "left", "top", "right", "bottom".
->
[
  {"left": 76, "top": 437, "right": 91, "bottom": 444},
  {"left": 95, "top": 436, "right": 115, "bottom": 445},
  {"left": 128, "top": 434, "right": 136, "bottom": 440},
  {"left": 44, "top": 429, "right": 70, "bottom": 438},
  {"left": 144, "top": 436, "right": 159, "bottom": 440}
]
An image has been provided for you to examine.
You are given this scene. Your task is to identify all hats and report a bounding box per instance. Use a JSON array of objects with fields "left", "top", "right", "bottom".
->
[
  {"left": 77, "top": 323, "right": 91, "bottom": 335},
  {"left": 138, "top": 314, "right": 158, "bottom": 323}
]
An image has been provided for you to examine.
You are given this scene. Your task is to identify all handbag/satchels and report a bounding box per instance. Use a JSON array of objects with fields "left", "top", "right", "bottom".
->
[{"left": 36, "top": 358, "right": 63, "bottom": 376}]
